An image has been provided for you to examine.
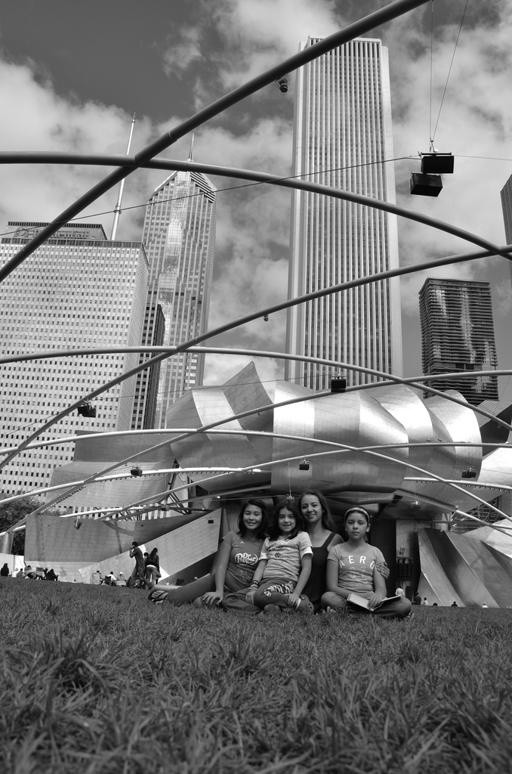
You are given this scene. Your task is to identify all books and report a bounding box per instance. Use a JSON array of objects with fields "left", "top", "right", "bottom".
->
[{"left": 346, "top": 588, "right": 402, "bottom": 614}]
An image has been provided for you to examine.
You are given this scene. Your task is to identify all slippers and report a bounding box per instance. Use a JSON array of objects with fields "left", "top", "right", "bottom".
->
[{"left": 149, "top": 590, "right": 168, "bottom": 601}]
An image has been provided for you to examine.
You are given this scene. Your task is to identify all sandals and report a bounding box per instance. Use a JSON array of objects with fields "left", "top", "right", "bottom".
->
[{"left": 295, "top": 594, "right": 314, "bottom": 612}]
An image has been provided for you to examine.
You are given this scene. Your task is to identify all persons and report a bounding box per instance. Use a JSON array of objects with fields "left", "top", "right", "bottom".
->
[
  {"left": 146, "top": 500, "right": 267, "bottom": 610},
  {"left": 396, "top": 586, "right": 490, "bottom": 609},
  {"left": 1, "top": 542, "right": 160, "bottom": 589},
  {"left": 320, "top": 505, "right": 414, "bottom": 617},
  {"left": 223, "top": 504, "right": 315, "bottom": 616},
  {"left": 376, "top": 562, "right": 390, "bottom": 579},
  {"left": 295, "top": 487, "right": 345, "bottom": 610}
]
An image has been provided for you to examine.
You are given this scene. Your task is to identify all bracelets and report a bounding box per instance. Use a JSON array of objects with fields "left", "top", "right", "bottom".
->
[{"left": 251, "top": 579, "right": 259, "bottom": 586}]
